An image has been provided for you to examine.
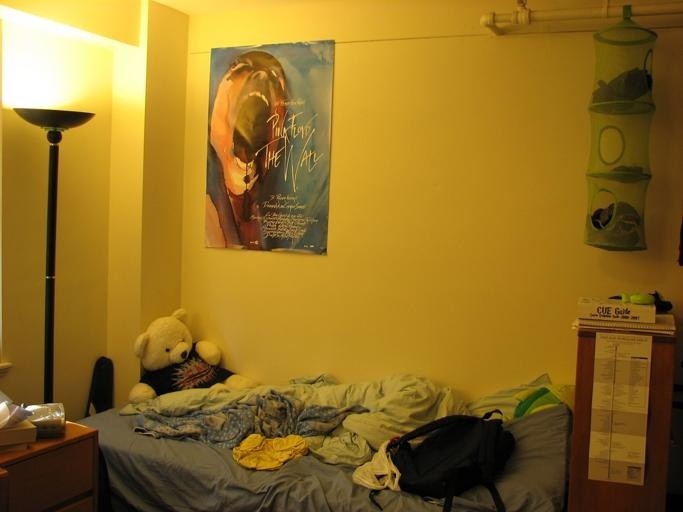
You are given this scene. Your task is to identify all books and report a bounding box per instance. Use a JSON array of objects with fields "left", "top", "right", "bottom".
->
[{"left": 571, "top": 303, "right": 677, "bottom": 337}]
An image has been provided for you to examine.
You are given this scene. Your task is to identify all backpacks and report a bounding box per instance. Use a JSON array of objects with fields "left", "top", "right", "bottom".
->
[{"left": 369, "top": 409, "right": 515, "bottom": 512}]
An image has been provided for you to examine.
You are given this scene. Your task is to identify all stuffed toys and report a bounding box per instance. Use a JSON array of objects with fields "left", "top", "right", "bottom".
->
[{"left": 128, "top": 308, "right": 257, "bottom": 405}]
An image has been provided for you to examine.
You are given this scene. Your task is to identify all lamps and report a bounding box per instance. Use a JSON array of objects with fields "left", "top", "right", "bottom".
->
[{"left": 8, "top": 106, "right": 97, "bottom": 403}]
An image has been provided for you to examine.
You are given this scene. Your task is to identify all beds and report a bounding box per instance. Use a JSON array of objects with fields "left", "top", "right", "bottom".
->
[{"left": 67, "top": 371, "right": 572, "bottom": 512}]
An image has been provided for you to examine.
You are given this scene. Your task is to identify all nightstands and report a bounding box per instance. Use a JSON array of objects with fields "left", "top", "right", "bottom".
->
[{"left": 0, "top": 417, "right": 102, "bottom": 512}]
